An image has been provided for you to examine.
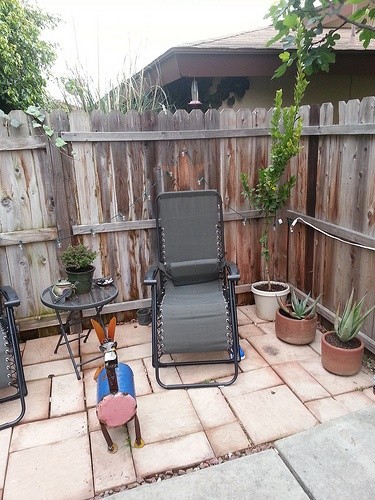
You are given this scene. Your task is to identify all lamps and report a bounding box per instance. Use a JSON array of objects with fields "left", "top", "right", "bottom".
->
[{"left": 187, "top": 76, "right": 203, "bottom": 111}]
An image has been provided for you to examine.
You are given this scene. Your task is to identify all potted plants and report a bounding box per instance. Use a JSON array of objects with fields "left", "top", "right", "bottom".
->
[
  {"left": 275, "top": 288, "right": 325, "bottom": 346},
  {"left": 61, "top": 243, "right": 97, "bottom": 295},
  {"left": 239, "top": 17, "right": 311, "bottom": 323},
  {"left": 322, "top": 286, "right": 375, "bottom": 376}
]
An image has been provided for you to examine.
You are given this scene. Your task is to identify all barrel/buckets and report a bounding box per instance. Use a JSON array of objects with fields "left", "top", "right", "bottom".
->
[{"left": 136, "top": 308, "right": 151, "bottom": 326}]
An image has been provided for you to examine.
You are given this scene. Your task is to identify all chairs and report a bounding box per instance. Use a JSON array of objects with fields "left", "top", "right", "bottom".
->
[
  {"left": 143, "top": 188, "right": 242, "bottom": 390},
  {"left": 0, "top": 284, "right": 28, "bottom": 432}
]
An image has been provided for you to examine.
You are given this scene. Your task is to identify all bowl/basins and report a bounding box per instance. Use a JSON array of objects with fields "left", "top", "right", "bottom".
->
[{"left": 95, "top": 278, "right": 113, "bottom": 286}]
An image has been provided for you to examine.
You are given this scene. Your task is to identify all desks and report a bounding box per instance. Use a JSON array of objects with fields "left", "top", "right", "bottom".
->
[{"left": 39, "top": 279, "right": 119, "bottom": 380}]
